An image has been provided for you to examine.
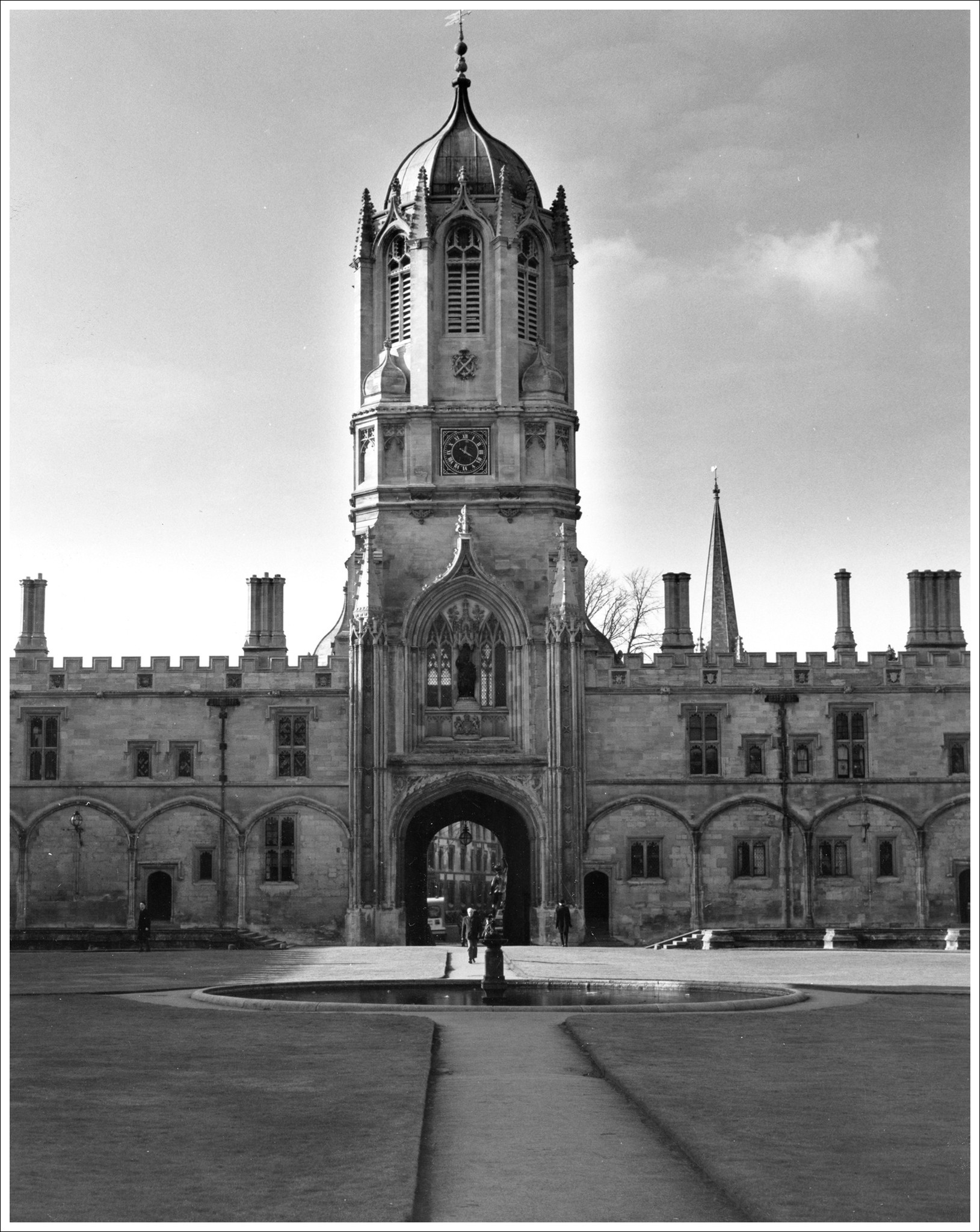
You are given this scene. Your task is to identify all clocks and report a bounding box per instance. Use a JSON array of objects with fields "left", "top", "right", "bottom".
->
[{"left": 441, "top": 427, "right": 491, "bottom": 476}]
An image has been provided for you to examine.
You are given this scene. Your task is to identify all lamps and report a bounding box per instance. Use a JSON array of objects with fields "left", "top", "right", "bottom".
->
[
  {"left": 70, "top": 811, "right": 83, "bottom": 832},
  {"left": 460, "top": 820, "right": 472, "bottom": 846}
]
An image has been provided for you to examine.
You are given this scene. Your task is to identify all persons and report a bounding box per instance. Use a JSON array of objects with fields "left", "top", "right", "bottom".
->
[
  {"left": 485, "top": 914, "right": 495, "bottom": 936},
  {"left": 461, "top": 908, "right": 482, "bottom": 962},
  {"left": 556, "top": 900, "right": 571, "bottom": 947},
  {"left": 137, "top": 901, "right": 151, "bottom": 952},
  {"left": 455, "top": 644, "right": 476, "bottom": 696}
]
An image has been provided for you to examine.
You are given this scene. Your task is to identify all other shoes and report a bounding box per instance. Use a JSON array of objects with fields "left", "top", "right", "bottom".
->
[
  {"left": 472, "top": 958, "right": 475, "bottom": 963},
  {"left": 469, "top": 960, "right": 472, "bottom": 963}
]
